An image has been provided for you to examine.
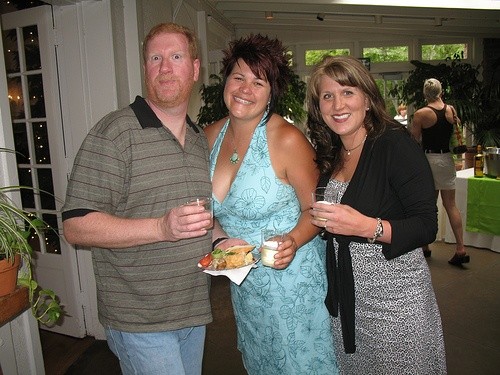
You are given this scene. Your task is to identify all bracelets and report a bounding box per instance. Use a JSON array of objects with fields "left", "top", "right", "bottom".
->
[
  {"left": 367, "top": 218, "right": 384, "bottom": 244},
  {"left": 211, "top": 237, "right": 230, "bottom": 251}
]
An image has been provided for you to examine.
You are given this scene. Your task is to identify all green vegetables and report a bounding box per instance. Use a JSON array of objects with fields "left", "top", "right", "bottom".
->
[{"left": 212, "top": 249, "right": 238, "bottom": 256}]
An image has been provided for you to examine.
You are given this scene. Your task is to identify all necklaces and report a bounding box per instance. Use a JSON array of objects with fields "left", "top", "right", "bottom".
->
[
  {"left": 342, "top": 134, "right": 366, "bottom": 161},
  {"left": 225, "top": 124, "right": 256, "bottom": 165}
]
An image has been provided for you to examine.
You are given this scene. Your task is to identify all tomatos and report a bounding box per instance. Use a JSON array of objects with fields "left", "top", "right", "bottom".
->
[{"left": 199, "top": 253, "right": 212, "bottom": 267}]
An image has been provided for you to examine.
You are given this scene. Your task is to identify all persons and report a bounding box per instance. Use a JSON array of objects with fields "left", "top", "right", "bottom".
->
[
  {"left": 308, "top": 55, "right": 447, "bottom": 375},
  {"left": 203, "top": 35, "right": 338, "bottom": 375},
  {"left": 61, "top": 22, "right": 250, "bottom": 375},
  {"left": 410, "top": 79, "right": 470, "bottom": 266},
  {"left": 394, "top": 104, "right": 408, "bottom": 128}
]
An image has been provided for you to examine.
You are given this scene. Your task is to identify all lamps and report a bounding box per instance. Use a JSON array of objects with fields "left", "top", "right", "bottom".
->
[
  {"left": 434, "top": 17, "right": 442, "bottom": 26},
  {"left": 265, "top": 12, "right": 272, "bottom": 19},
  {"left": 316, "top": 13, "right": 325, "bottom": 22},
  {"left": 374, "top": 16, "right": 383, "bottom": 25}
]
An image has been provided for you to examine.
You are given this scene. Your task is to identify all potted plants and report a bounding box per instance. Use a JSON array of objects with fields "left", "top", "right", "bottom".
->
[{"left": 0, "top": 148, "right": 69, "bottom": 328}]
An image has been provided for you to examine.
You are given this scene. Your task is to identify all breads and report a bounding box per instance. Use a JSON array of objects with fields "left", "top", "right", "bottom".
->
[{"left": 225, "top": 244, "right": 256, "bottom": 254}]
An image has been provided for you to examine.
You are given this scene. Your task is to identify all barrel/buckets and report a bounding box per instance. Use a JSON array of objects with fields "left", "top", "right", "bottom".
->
[{"left": 485, "top": 148, "right": 500, "bottom": 177}]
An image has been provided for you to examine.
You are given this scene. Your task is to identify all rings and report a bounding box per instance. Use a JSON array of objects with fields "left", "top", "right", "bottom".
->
[{"left": 324, "top": 221, "right": 327, "bottom": 228}]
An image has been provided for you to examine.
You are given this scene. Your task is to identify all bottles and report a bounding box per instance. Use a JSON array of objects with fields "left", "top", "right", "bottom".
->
[{"left": 474, "top": 145, "right": 484, "bottom": 177}]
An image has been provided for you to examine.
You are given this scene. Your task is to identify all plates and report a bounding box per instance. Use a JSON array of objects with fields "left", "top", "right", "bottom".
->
[{"left": 197, "top": 251, "right": 261, "bottom": 270}]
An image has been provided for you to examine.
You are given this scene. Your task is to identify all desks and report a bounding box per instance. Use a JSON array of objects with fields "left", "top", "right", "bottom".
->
[{"left": 436, "top": 167, "right": 500, "bottom": 254}]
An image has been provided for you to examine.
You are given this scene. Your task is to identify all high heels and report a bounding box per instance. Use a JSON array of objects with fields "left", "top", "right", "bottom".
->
[
  {"left": 424, "top": 250, "right": 431, "bottom": 257},
  {"left": 447, "top": 253, "right": 473, "bottom": 265}
]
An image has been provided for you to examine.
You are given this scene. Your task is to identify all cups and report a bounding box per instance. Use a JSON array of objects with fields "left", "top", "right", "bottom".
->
[
  {"left": 184, "top": 197, "right": 214, "bottom": 229},
  {"left": 260, "top": 229, "right": 287, "bottom": 268},
  {"left": 311, "top": 187, "right": 337, "bottom": 223}
]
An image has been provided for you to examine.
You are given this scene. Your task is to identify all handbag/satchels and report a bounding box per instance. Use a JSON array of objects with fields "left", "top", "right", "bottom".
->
[{"left": 453, "top": 104, "right": 474, "bottom": 155}]
